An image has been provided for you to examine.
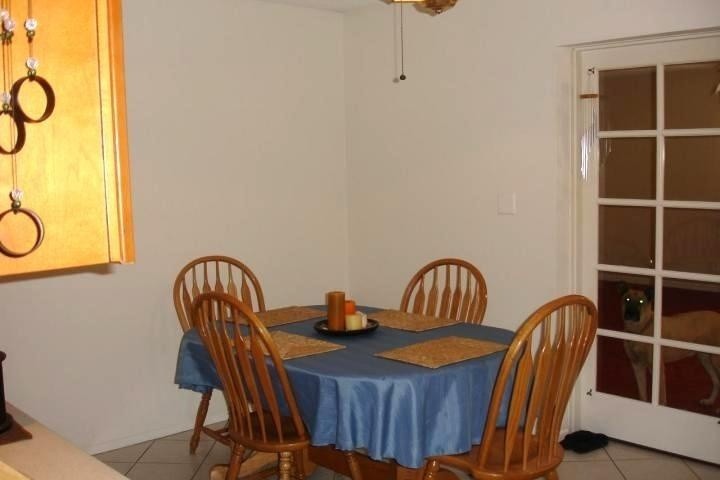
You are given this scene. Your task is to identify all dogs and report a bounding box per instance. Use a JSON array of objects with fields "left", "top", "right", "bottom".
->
[{"left": 616, "top": 280, "right": 720, "bottom": 406}]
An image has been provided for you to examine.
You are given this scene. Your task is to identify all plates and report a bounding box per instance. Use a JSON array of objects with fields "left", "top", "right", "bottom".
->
[{"left": 313, "top": 318, "right": 379, "bottom": 336}]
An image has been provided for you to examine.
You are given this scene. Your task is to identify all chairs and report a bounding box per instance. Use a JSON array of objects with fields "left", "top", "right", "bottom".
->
[
  {"left": 400, "top": 258, "right": 487, "bottom": 325},
  {"left": 191, "top": 291, "right": 365, "bottom": 480},
  {"left": 424, "top": 295, "right": 598, "bottom": 480},
  {"left": 174, "top": 256, "right": 266, "bottom": 464}
]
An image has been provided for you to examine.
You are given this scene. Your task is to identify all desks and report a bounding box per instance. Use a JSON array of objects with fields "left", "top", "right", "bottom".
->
[{"left": 0, "top": 399, "right": 131, "bottom": 480}]
